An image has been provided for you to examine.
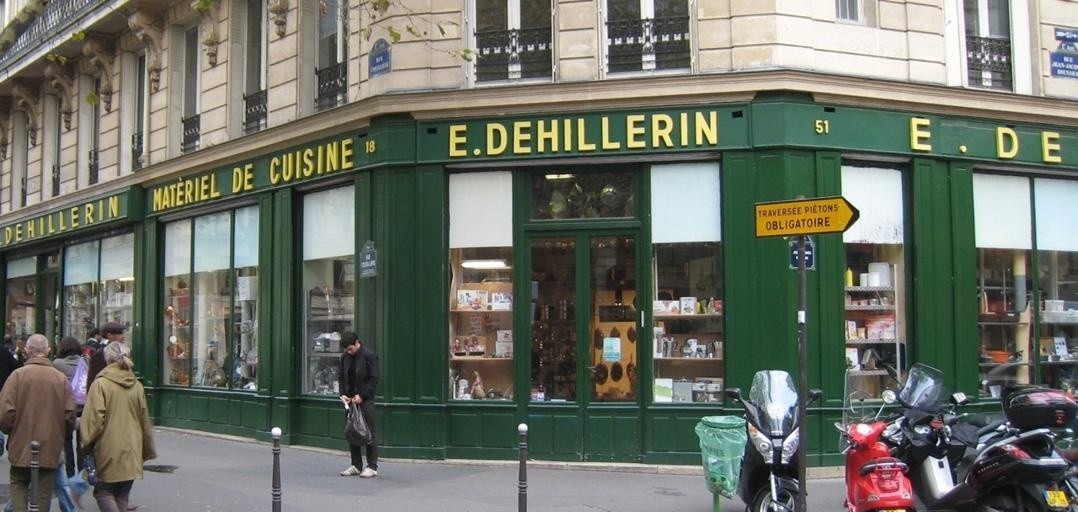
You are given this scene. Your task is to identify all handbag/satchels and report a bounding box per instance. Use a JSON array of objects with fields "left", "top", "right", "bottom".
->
[{"left": 345, "top": 403, "right": 372, "bottom": 445}]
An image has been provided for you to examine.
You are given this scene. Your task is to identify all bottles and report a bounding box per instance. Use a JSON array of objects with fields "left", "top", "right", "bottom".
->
[{"left": 846, "top": 267, "right": 853, "bottom": 287}]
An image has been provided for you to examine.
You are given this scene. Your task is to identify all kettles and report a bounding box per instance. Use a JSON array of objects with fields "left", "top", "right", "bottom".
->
[
  {"left": 679, "top": 344, "right": 693, "bottom": 358},
  {"left": 706, "top": 342, "right": 716, "bottom": 358}
]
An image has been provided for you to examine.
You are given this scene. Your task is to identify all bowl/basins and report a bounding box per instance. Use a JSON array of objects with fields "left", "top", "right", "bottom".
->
[{"left": 851, "top": 296, "right": 891, "bottom": 306}]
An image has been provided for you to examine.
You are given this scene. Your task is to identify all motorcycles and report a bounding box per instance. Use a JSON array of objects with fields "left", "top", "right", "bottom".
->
[{"left": 720, "top": 368, "right": 823, "bottom": 510}]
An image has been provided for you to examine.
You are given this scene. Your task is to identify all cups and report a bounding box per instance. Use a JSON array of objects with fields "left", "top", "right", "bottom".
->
[
  {"left": 867, "top": 272, "right": 881, "bottom": 287},
  {"left": 686, "top": 338, "right": 698, "bottom": 357},
  {"left": 859, "top": 273, "right": 868, "bottom": 288}
]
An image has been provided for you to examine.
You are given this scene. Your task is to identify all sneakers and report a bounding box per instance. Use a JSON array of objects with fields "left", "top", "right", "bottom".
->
[{"left": 340, "top": 465, "right": 378, "bottom": 477}]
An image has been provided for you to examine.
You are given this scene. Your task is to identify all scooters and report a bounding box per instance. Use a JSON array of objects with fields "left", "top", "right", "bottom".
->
[
  {"left": 998, "top": 384, "right": 1078, "bottom": 493},
  {"left": 833, "top": 369, "right": 916, "bottom": 512},
  {"left": 885, "top": 360, "right": 1074, "bottom": 512}
]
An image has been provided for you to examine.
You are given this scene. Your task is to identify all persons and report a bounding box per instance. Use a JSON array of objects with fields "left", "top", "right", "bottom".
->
[
  {"left": 338, "top": 331, "right": 381, "bottom": 478},
  {"left": 0, "top": 321, "right": 157, "bottom": 512}
]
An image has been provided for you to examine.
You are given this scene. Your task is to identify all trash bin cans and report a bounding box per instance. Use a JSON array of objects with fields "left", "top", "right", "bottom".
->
[{"left": 695, "top": 415, "right": 748, "bottom": 512}]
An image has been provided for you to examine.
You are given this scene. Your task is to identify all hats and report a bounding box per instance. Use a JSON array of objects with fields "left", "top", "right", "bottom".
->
[{"left": 102, "top": 323, "right": 126, "bottom": 332}]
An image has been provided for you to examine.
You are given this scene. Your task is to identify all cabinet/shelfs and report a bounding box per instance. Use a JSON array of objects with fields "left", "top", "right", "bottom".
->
[
  {"left": 448, "top": 282, "right": 516, "bottom": 400},
  {"left": 978, "top": 283, "right": 1032, "bottom": 382},
  {"left": 843, "top": 283, "right": 902, "bottom": 402},
  {"left": 1038, "top": 303, "right": 1078, "bottom": 401},
  {"left": 653, "top": 307, "right": 724, "bottom": 403},
  {"left": 64, "top": 288, "right": 353, "bottom": 398}
]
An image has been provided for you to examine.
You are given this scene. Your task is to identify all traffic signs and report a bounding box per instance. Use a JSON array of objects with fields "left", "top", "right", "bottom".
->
[{"left": 755, "top": 195, "right": 860, "bottom": 237}]
